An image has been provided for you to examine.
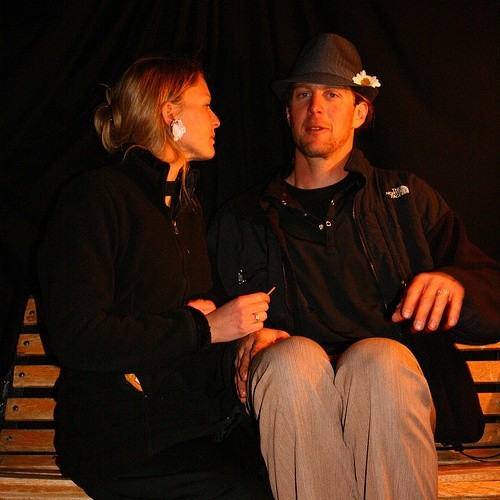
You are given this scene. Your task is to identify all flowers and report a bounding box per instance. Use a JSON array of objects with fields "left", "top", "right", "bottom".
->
[{"left": 352, "top": 69, "right": 381, "bottom": 88}]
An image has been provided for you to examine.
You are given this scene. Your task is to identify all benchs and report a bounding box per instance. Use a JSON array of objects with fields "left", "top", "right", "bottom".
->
[{"left": 0, "top": 297, "right": 500, "bottom": 500}]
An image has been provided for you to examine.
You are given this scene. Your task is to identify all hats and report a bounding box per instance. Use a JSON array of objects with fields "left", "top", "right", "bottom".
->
[{"left": 271, "top": 33, "right": 379, "bottom": 101}]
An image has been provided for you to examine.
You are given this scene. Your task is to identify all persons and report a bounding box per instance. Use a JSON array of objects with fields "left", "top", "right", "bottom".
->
[
  {"left": 203, "top": 31, "right": 500, "bottom": 500},
  {"left": 27, "top": 54, "right": 270, "bottom": 500}
]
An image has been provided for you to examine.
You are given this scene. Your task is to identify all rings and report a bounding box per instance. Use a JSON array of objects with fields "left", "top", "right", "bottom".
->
[
  {"left": 252, "top": 313, "right": 260, "bottom": 324},
  {"left": 436, "top": 288, "right": 449, "bottom": 295}
]
